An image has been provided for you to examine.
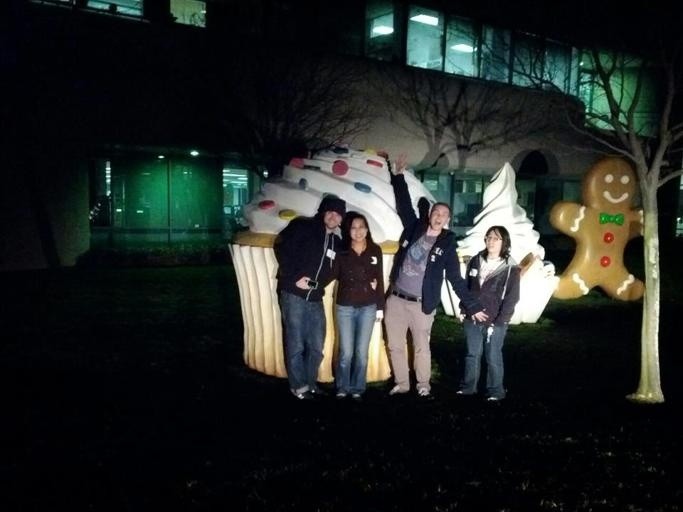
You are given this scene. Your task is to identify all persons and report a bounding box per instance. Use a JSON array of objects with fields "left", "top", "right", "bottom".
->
[
  {"left": 383, "top": 154, "right": 489, "bottom": 402},
  {"left": 272, "top": 195, "right": 378, "bottom": 401},
  {"left": 332, "top": 213, "right": 386, "bottom": 401},
  {"left": 455, "top": 225, "right": 520, "bottom": 402}
]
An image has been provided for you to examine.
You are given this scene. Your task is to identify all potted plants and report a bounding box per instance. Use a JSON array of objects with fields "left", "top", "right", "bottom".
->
[
  {"left": 134, "top": 242, "right": 171, "bottom": 267},
  {"left": 117, "top": 246, "right": 134, "bottom": 267},
  {"left": 174, "top": 240, "right": 215, "bottom": 266}
]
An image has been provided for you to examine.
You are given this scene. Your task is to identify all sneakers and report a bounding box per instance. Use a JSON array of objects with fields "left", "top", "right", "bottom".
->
[
  {"left": 335, "top": 392, "right": 347, "bottom": 401},
  {"left": 390, "top": 384, "right": 409, "bottom": 395},
  {"left": 417, "top": 388, "right": 430, "bottom": 397},
  {"left": 487, "top": 397, "right": 500, "bottom": 401},
  {"left": 292, "top": 389, "right": 329, "bottom": 400},
  {"left": 352, "top": 393, "right": 364, "bottom": 402}
]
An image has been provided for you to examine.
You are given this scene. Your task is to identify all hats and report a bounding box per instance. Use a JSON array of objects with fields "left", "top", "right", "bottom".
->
[{"left": 324, "top": 199, "right": 345, "bottom": 217}]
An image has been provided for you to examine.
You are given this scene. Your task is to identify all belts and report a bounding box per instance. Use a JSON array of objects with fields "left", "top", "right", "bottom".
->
[{"left": 392, "top": 291, "right": 423, "bottom": 302}]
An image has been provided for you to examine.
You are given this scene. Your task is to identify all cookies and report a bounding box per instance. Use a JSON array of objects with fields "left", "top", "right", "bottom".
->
[{"left": 549, "top": 158, "right": 645, "bottom": 301}]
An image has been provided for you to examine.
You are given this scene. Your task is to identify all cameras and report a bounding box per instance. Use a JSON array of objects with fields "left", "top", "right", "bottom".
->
[{"left": 306, "top": 280, "right": 318, "bottom": 289}]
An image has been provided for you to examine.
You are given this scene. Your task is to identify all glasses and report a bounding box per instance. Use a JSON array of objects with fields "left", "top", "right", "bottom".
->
[{"left": 485, "top": 236, "right": 502, "bottom": 241}]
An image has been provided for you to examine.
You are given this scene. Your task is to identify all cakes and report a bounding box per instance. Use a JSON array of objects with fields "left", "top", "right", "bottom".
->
[
  {"left": 229, "top": 137, "right": 451, "bottom": 384},
  {"left": 441, "top": 162, "right": 560, "bottom": 326}
]
